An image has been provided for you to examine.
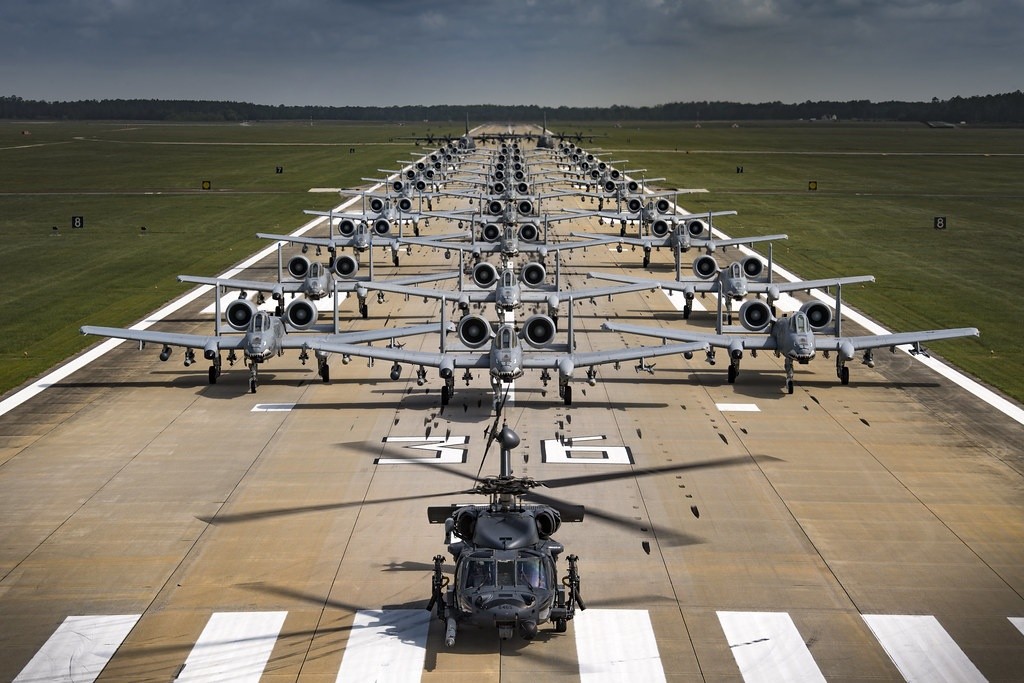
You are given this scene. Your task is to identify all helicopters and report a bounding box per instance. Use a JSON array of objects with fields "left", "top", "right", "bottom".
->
[{"left": 426, "top": 380, "right": 585, "bottom": 647}]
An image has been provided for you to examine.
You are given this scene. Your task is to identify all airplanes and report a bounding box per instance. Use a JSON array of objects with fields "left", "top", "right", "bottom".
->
[{"left": 78, "top": 111, "right": 980, "bottom": 416}]
